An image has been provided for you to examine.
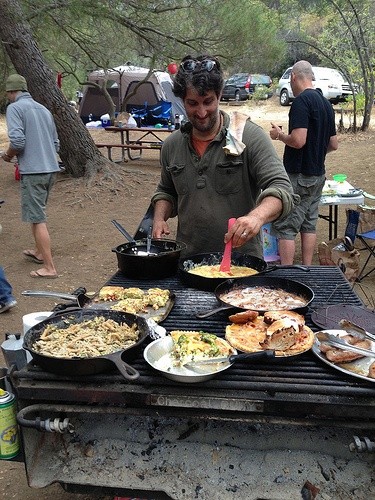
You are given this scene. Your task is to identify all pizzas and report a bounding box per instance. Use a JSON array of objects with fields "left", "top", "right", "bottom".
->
[
  {"left": 225, "top": 316, "right": 314, "bottom": 356},
  {"left": 169, "top": 330, "right": 234, "bottom": 365}
]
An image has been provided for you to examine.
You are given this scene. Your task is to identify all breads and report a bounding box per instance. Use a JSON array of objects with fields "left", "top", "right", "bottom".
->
[
  {"left": 228, "top": 310, "right": 305, "bottom": 350},
  {"left": 100, "top": 286, "right": 169, "bottom": 315}
]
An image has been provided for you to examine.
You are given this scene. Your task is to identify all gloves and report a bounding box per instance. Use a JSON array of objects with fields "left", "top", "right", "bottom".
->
[
  {"left": 0, "top": 152, "right": 15, "bottom": 162},
  {"left": 56, "top": 153, "right": 66, "bottom": 170}
]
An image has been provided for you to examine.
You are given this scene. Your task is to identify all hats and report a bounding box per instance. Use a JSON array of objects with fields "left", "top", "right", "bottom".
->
[{"left": 6, "top": 74, "right": 28, "bottom": 92}]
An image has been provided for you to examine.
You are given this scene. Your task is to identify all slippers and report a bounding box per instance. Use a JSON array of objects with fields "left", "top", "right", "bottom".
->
[
  {"left": 23, "top": 250, "right": 44, "bottom": 264},
  {"left": 30, "top": 268, "right": 58, "bottom": 279}
]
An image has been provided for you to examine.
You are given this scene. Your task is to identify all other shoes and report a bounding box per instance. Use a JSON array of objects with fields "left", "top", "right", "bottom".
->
[{"left": 0, "top": 300, "right": 17, "bottom": 314}]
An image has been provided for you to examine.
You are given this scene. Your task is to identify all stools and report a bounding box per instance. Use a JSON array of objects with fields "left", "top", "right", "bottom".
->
[{"left": 355, "top": 229, "right": 375, "bottom": 283}]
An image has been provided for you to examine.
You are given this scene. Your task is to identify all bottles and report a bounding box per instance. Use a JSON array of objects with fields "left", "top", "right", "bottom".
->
[
  {"left": 0, "top": 389, "right": 20, "bottom": 460},
  {"left": 168, "top": 113, "right": 186, "bottom": 130}
]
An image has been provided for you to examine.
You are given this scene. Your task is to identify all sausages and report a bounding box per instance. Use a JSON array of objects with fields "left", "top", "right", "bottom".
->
[{"left": 320, "top": 331, "right": 375, "bottom": 379}]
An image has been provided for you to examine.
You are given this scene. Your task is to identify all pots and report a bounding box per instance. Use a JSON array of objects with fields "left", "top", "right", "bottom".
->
[
  {"left": 21, "top": 289, "right": 175, "bottom": 324},
  {"left": 196, "top": 275, "right": 314, "bottom": 318},
  {"left": 178, "top": 252, "right": 310, "bottom": 289},
  {"left": 23, "top": 309, "right": 150, "bottom": 380},
  {"left": 108, "top": 218, "right": 187, "bottom": 280}
]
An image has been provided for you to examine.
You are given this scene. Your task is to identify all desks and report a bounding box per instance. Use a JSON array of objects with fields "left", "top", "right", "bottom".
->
[
  {"left": 105, "top": 128, "right": 175, "bottom": 162},
  {"left": 318, "top": 180, "right": 364, "bottom": 241}
]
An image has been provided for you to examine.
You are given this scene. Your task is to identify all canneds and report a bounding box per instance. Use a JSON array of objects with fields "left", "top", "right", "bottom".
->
[{"left": 118, "top": 121, "right": 122, "bottom": 128}]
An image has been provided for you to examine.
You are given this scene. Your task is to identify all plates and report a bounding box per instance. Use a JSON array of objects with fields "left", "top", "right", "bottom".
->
[
  {"left": 312, "top": 329, "right": 375, "bottom": 382},
  {"left": 226, "top": 325, "right": 315, "bottom": 358}
]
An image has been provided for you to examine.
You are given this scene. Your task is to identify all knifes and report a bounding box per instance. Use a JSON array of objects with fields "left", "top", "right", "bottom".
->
[
  {"left": 147, "top": 225, "right": 153, "bottom": 253},
  {"left": 183, "top": 349, "right": 275, "bottom": 367}
]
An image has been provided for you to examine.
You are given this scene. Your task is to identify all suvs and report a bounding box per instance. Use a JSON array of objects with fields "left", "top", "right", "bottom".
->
[
  {"left": 221, "top": 72, "right": 273, "bottom": 101},
  {"left": 275, "top": 66, "right": 363, "bottom": 105}
]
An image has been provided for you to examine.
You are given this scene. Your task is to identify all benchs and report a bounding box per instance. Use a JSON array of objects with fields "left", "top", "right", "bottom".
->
[{"left": 95, "top": 140, "right": 162, "bottom": 165}]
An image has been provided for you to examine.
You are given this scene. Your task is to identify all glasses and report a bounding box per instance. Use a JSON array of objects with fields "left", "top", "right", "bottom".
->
[{"left": 180, "top": 60, "right": 216, "bottom": 72}]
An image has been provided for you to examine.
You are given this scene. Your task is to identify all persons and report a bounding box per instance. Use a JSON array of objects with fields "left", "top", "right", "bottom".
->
[
  {"left": 151, "top": 55, "right": 298, "bottom": 259},
  {"left": 0, "top": 265, "right": 17, "bottom": 314},
  {"left": 0, "top": 74, "right": 62, "bottom": 279},
  {"left": 270, "top": 61, "right": 338, "bottom": 265}
]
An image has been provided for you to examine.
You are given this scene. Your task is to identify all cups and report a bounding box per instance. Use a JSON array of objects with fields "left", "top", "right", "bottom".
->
[{"left": 271, "top": 125, "right": 283, "bottom": 131}]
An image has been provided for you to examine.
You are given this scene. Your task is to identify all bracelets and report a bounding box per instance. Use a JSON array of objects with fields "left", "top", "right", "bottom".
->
[{"left": 275, "top": 131, "right": 282, "bottom": 140}]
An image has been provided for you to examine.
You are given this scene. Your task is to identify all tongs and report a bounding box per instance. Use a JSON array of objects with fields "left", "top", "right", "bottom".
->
[{"left": 315, "top": 319, "right": 375, "bottom": 358}]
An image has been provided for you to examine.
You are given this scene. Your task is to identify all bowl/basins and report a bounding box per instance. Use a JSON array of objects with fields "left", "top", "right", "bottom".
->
[
  {"left": 333, "top": 174, "right": 347, "bottom": 183},
  {"left": 143, "top": 333, "right": 238, "bottom": 383},
  {"left": 126, "top": 123, "right": 137, "bottom": 128}
]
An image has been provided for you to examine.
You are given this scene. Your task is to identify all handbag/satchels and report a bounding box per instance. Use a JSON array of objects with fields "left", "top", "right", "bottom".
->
[{"left": 318, "top": 236, "right": 361, "bottom": 282}]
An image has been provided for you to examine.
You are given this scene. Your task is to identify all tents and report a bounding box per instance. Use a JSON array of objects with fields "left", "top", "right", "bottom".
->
[{"left": 79, "top": 61, "right": 189, "bottom": 120}]
